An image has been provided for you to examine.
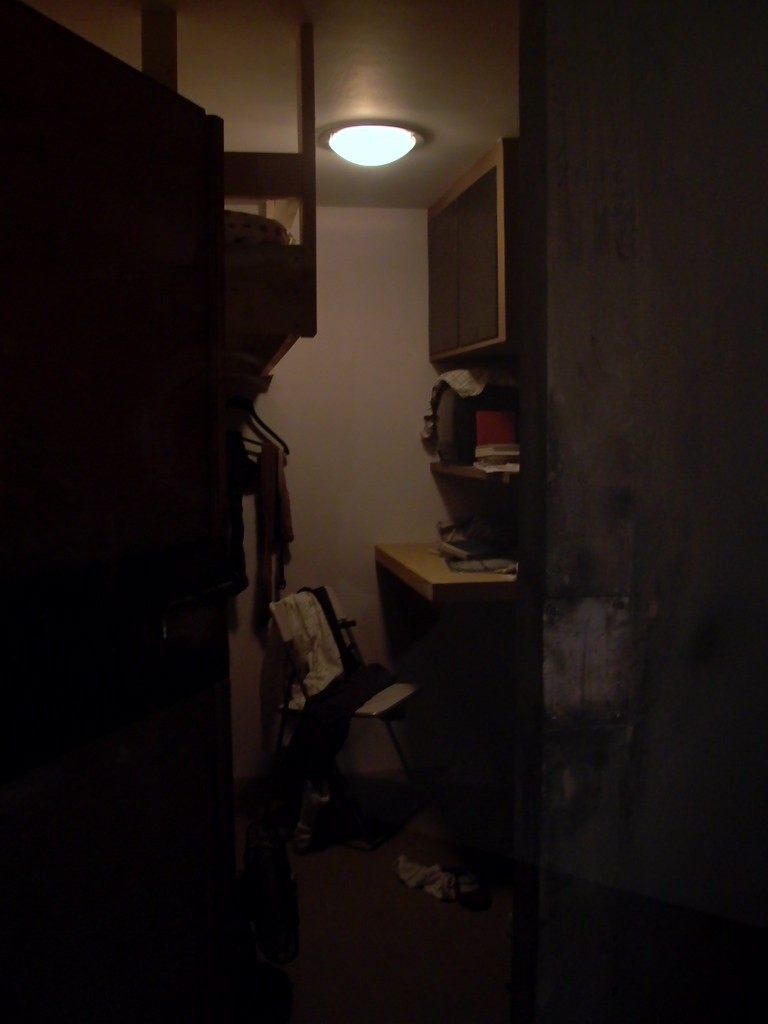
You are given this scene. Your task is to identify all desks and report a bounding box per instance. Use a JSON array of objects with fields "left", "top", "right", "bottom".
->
[{"left": 375, "top": 542, "right": 518, "bottom": 604}]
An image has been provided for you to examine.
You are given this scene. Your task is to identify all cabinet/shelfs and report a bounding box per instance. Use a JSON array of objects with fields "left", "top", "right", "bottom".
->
[{"left": 426, "top": 137, "right": 523, "bottom": 360}]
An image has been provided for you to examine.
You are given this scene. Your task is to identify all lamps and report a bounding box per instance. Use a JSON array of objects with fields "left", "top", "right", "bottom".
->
[{"left": 320, "top": 122, "right": 426, "bottom": 167}]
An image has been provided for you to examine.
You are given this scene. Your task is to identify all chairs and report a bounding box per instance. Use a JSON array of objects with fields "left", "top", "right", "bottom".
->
[{"left": 268, "top": 585, "right": 426, "bottom": 850}]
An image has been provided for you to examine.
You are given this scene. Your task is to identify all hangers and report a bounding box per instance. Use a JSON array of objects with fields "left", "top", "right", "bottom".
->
[{"left": 223, "top": 388, "right": 291, "bottom": 468}]
